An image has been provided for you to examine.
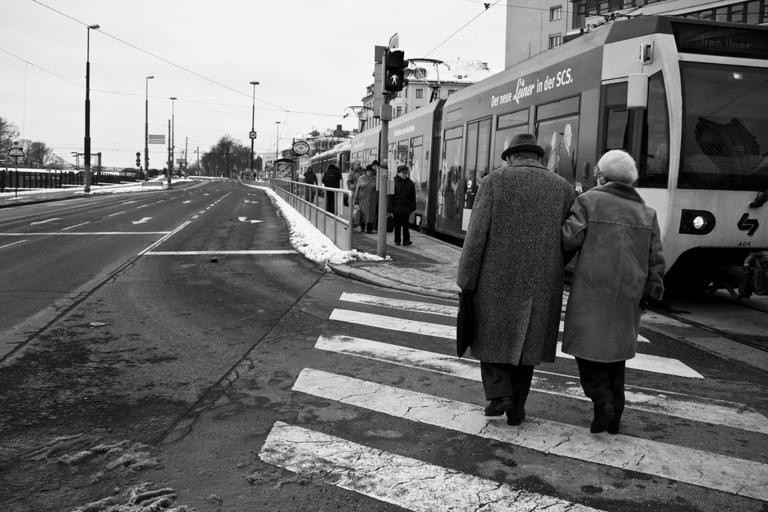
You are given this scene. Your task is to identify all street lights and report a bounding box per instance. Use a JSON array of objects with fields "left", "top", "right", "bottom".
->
[
  {"left": 169, "top": 96, "right": 179, "bottom": 177},
  {"left": 143, "top": 75, "right": 155, "bottom": 181},
  {"left": 249, "top": 80, "right": 259, "bottom": 179},
  {"left": 275, "top": 121, "right": 282, "bottom": 160},
  {"left": 84, "top": 23, "right": 102, "bottom": 193}
]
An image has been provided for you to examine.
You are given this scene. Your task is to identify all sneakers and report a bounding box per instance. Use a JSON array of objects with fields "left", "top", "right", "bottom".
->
[{"left": 396, "top": 241, "right": 412, "bottom": 246}]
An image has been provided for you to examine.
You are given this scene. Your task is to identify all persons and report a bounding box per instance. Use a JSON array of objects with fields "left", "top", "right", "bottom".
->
[
  {"left": 560, "top": 149, "right": 665, "bottom": 434},
  {"left": 346, "top": 159, "right": 365, "bottom": 228},
  {"left": 303, "top": 166, "right": 318, "bottom": 203},
  {"left": 322, "top": 162, "right": 343, "bottom": 213},
  {"left": 386, "top": 166, "right": 417, "bottom": 246},
  {"left": 546, "top": 131, "right": 559, "bottom": 172},
  {"left": 371, "top": 160, "right": 377, "bottom": 230},
  {"left": 560, "top": 123, "right": 573, "bottom": 179},
  {"left": 351, "top": 165, "right": 376, "bottom": 235},
  {"left": 456, "top": 134, "right": 574, "bottom": 426}
]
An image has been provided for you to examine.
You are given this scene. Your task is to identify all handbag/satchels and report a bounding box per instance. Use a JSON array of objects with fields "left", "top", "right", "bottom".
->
[
  {"left": 387, "top": 215, "right": 394, "bottom": 233},
  {"left": 456, "top": 290, "right": 475, "bottom": 358},
  {"left": 352, "top": 205, "right": 360, "bottom": 229}
]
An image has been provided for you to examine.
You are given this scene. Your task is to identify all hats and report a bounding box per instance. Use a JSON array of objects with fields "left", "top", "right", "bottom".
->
[
  {"left": 598, "top": 150, "right": 639, "bottom": 185},
  {"left": 501, "top": 133, "right": 545, "bottom": 161},
  {"left": 365, "top": 160, "right": 378, "bottom": 172},
  {"left": 397, "top": 166, "right": 409, "bottom": 173}
]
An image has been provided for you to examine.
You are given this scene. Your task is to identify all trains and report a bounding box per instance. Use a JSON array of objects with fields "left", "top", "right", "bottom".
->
[{"left": 307, "top": 11, "right": 767, "bottom": 302}]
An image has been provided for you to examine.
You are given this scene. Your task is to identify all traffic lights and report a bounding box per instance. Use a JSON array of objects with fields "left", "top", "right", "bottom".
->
[{"left": 385, "top": 49, "right": 407, "bottom": 91}]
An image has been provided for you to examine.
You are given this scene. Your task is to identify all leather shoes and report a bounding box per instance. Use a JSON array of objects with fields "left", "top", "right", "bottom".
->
[
  {"left": 484, "top": 395, "right": 525, "bottom": 426},
  {"left": 590, "top": 402, "right": 621, "bottom": 435}
]
